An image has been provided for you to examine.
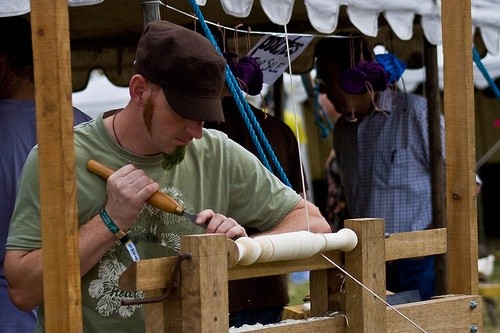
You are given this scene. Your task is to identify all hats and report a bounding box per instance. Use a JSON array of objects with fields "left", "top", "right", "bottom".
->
[{"left": 132, "top": 21, "right": 226, "bottom": 122}]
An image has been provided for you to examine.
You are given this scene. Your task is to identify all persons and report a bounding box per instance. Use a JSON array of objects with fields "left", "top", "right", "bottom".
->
[
  {"left": 314, "top": 33, "right": 482, "bottom": 299},
  {"left": 0, "top": 16, "right": 332, "bottom": 333}
]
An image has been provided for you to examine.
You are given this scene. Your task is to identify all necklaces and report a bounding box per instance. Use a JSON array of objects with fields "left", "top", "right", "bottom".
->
[{"left": 112, "top": 108, "right": 123, "bottom": 147}]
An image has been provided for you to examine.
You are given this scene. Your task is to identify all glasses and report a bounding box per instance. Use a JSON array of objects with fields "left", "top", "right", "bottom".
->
[{"left": 314, "top": 76, "right": 325, "bottom": 86}]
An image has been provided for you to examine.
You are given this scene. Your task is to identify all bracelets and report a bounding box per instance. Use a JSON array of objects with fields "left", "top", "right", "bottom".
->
[{"left": 99, "top": 208, "right": 140, "bottom": 262}]
